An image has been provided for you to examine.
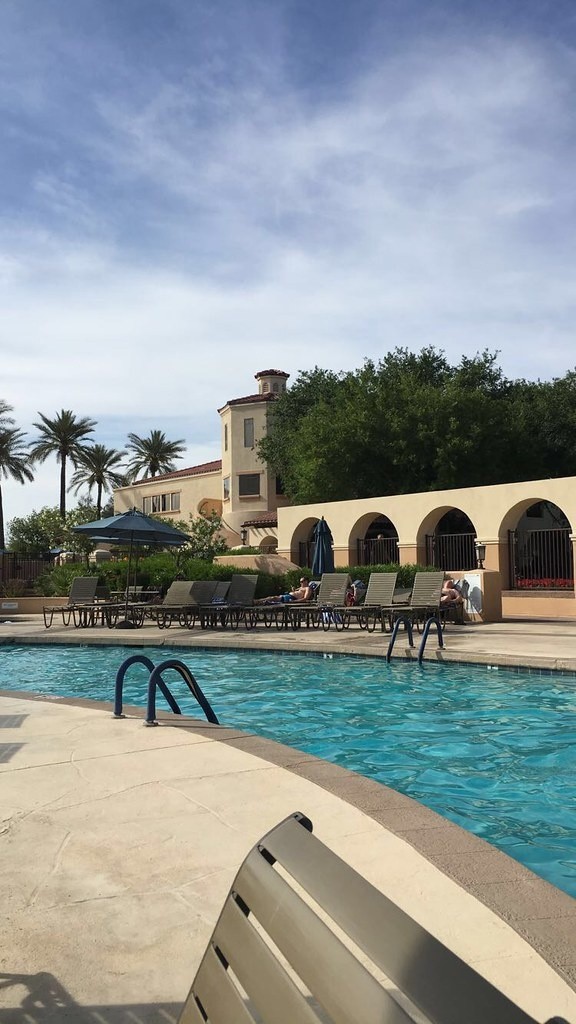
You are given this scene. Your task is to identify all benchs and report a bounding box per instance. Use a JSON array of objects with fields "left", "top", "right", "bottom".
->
[{"left": 175, "top": 812, "right": 571, "bottom": 1024}]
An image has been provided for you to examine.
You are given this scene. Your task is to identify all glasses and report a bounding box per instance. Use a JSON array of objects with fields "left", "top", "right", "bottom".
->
[{"left": 300, "top": 581, "right": 305, "bottom": 583}]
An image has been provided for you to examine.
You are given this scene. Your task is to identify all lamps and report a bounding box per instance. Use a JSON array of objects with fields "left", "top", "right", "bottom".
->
[
  {"left": 475, "top": 542, "right": 486, "bottom": 570},
  {"left": 240, "top": 529, "right": 247, "bottom": 545}
]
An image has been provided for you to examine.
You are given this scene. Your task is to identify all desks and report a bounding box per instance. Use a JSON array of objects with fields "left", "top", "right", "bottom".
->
[{"left": 109, "top": 591, "right": 159, "bottom": 603}]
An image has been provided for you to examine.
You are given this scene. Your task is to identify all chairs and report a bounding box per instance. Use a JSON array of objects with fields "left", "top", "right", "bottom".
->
[{"left": 43, "top": 571, "right": 464, "bottom": 634}]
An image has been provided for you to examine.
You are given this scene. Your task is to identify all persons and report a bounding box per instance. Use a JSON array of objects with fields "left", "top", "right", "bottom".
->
[
  {"left": 432, "top": 580, "right": 462, "bottom": 606},
  {"left": 331, "top": 584, "right": 357, "bottom": 606},
  {"left": 250, "top": 577, "right": 312, "bottom": 603}
]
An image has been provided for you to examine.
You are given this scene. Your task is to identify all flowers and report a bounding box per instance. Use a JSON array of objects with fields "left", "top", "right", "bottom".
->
[{"left": 517, "top": 579, "right": 574, "bottom": 590}]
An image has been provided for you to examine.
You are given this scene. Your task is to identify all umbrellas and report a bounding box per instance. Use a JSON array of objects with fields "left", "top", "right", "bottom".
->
[
  {"left": 72, "top": 506, "right": 192, "bottom": 622},
  {"left": 87, "top": 535, "right": 187, "bottom": 591},
  {"left": 311, "top": 516, "right": 335, "bottom": 577}
]
